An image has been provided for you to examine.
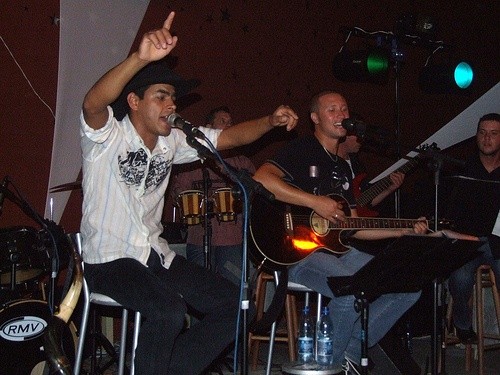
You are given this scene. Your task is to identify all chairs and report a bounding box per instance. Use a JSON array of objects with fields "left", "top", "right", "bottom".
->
[
  {"left": 74, "top": 232, "right": 150, "bottom": 375},
  {"left": 265, "top": 271, "right": 321, "bottom": 375}
]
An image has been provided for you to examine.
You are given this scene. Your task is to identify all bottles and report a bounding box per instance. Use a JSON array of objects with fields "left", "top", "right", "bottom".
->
[
  {"left": 317, "top": 306, "right": 333, "bottom": 365},
  {"left": 297, "top": 305, "right": 314, "bottom": 364},
  {"left": 405, "top": 322, "right": 412, "bottom": 354}
]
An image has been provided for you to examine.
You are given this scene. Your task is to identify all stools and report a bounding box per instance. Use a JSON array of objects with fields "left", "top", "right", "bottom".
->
[
  {"left": 247, "top": 272, "right": 298, "bottom": 372},
  {"left": 437, "top": 265, "right": 500, "bottom": 375}
]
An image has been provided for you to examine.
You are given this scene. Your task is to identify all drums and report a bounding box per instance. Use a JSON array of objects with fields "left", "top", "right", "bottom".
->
[
  {"left": 40, "top": 224, "right": 68, "bottom": 268},
  {"left": 0, "top": 225, "right": 47, "bottom": 290},
  {"left": 173, "top": 189, "right": 206, "bottom": 225},
  {"left": 0, "top": 297, "right": 80, "bottom": 375},
  {"left": 209, "top": 188, "right": 235, "bottom": 223}
]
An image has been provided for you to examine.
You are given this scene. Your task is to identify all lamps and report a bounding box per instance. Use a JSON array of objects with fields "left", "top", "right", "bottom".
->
[
  {"left": 419, "top": 59, "right": 475, "bottom": 99},
  {"left": 333, "top": 46, "right": 389, "bottom": 85}
]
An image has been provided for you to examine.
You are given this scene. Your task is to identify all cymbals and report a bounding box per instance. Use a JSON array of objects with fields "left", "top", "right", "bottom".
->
[{"left": 49, "top": 181, "right": 81, "bottom": 195}]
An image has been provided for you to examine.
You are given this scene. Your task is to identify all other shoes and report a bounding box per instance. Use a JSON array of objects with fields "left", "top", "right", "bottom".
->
[
  {"left": 454, "top": 324, "right": 478, "bottom": 345},
  {"left": 411, "top": 328, "right": 444, "bottom": 340}
]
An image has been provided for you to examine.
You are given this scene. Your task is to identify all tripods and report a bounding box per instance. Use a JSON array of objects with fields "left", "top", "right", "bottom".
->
[{"left": 76, "top": 305, "right": 130, "bottom": 375}]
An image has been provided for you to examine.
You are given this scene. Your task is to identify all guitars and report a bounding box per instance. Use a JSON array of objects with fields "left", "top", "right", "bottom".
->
[
  {"left": 246, "top": 179, "right": 453, "bottom": 272},
  {"left": 332, "top": 142, "right": 441, "bottom": 218}
]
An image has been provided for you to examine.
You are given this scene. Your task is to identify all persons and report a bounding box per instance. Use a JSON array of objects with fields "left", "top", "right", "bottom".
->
[
  {"left": 79, "top": 11, "right": 299, "bottom": 374},
  {"left": 172, "top": 107, "right": 255, "bottom": 375},
  {"left": 253, "top": 90, "right": 429, "bottom": 375},
  {"left": 449, "top": 113, "right": 500, "bottom": 344},
  {"left": 339, "top": 134, "right": 405, "bottom": 219}
]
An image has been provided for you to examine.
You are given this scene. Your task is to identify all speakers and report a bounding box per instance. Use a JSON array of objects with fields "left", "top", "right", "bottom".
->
[{"left": 365, "top": 330, "right": 421, "bottom": 375}]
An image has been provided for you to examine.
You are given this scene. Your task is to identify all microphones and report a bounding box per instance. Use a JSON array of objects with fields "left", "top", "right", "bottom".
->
[
  {"left": 0, "top": 176, "right": 8, "bottom": 215},
  {"left": 167, "top": 113, "right": 206, "bottom": 140},
  {"left": 341, "top": 118, "right": 388, "bottom": 138}
]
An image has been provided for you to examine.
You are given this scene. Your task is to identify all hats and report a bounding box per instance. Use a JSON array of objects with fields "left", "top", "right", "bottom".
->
[{"left": 123, "top": 62, "right": 176, "bottom": 97}]
